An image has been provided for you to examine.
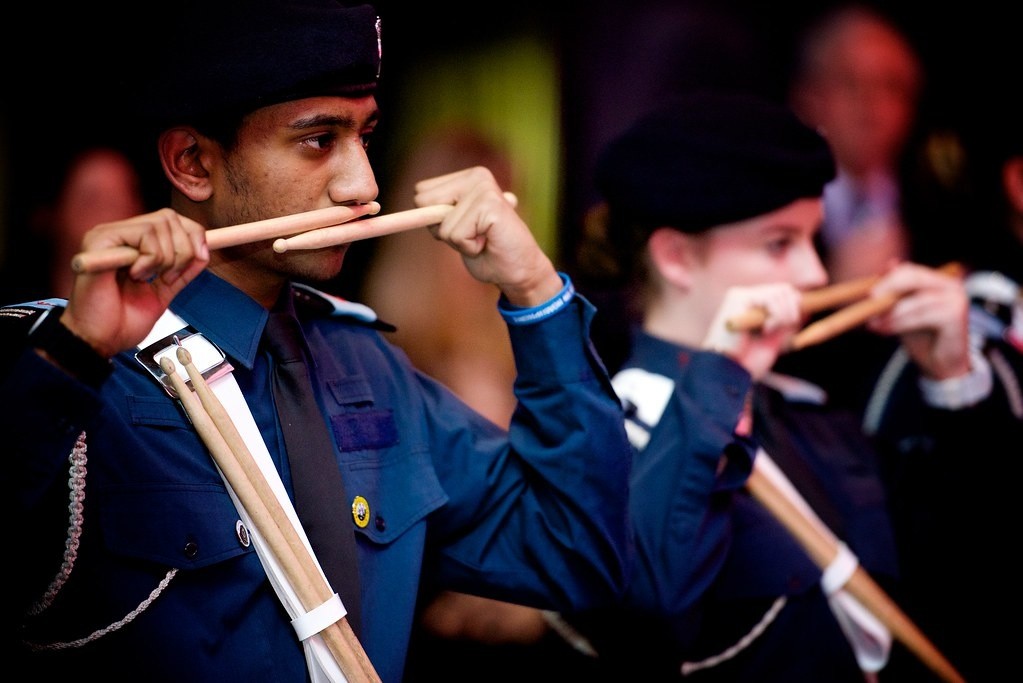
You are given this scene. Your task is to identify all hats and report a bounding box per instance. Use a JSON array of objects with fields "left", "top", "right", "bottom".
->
[
  {"left": 595, "top": 98, "right": 836, "bottom": 253},
  {"left": 139, "top": 0, "right": 383, "bottom": 125}
]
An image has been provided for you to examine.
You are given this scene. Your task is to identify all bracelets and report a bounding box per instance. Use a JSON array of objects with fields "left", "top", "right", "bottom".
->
[
  {"left": 496, "top": 270, "right": 575, "bottom": 326},
  {"left": 25, "top": 304, "right": 115, "bottom": 392}
]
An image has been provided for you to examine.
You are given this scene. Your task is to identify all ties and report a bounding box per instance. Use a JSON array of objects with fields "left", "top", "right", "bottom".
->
[
  {"left": 261, "top": 311, "right": 359, "bottom": 644},
  {"left": 849, "top": 175, "right": 871, "bottom": 228},
  {"left": 752, "top": 383, "right": 842, "bottom": 540}
]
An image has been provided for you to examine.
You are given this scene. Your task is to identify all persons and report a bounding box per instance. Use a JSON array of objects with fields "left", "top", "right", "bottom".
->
[
  {"left": 0, "top": 0, "right": 633, "bottom": 683},
  {"left": 550, "top": 10, "right": 1023, "bottom": 683}
]
